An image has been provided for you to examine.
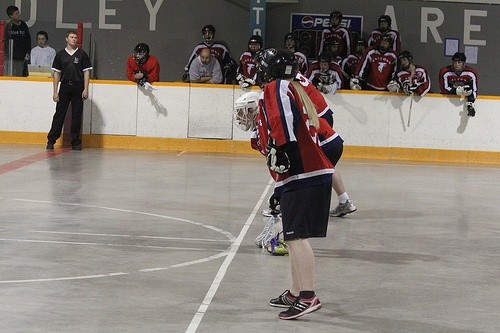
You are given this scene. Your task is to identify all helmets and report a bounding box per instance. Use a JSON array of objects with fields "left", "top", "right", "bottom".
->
[
  {"left": 247, "top": 36, "right": 264, "bottom": 56},
  {"left": 233, "top": 92, "right": 259, "bottom": 131},
  {"left": 452, "top": 52, "right": 467, "bottom": 75},
  {"left": 255, "top": 47, "right": 298, "bottom": 91},
  {"left": 284, "top": 33, "right": 299, "bottom": 46},
  {"left": 354, "top": 15, "right": 413, "bottom": 69},
  {"left": 318, "top": 11, "right": 343, "bottom": 71},
  {"left": 133, "top": 43, "right": 151, "bottom": 65},
  {"left": 202, "top": 25, "right": 216, "bottom": 43}
]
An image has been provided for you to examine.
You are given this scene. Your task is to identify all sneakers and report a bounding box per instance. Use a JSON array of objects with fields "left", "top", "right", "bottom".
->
[
  {"left": 267, "top": 239, "right": 290, "bottom": 256},
  {"left": 261, "top": 204, "right": 282, "bottom": 217},
  {"left": 278, "top": 293, "right": 321, "bottom": 320},
  {"left": 329, "top": 200, "right": 356, "bottom": 216},
  {"left": 73, "top": 143, "right": 82, "bottom": 150},
  {"left": 269, "top": 290, "right": 299, "bottom": 307},
  {"left": 47, "top": 142, "right": 55, "bottom": 149}
]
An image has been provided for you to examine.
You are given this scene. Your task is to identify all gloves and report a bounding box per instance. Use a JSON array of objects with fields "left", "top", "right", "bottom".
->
[
  {"left": 317, "top": 83, "right": 335, "bottom": 95},
  {"left": 350, "top": 78, "right": 361, "bottom": 90},
  {"left": 456, "top": 85, "right": 472, "bottom": 96},
  {"left": 466, "top": 102, "right": 475, "bottom": 117},
  {"left": 237, "top": 74, "right": 250, "bottom": 89},
  {"left": 388, "top": 81, "right": 400, "bottom": 93},
  {"left": 143, "top": 81, "right": 158, "bottom": 92}
]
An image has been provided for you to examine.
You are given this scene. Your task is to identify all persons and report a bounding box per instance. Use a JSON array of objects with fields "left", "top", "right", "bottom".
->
[
  {"left": 236, "top": 34, "right": 264, "bottom": 88},
  {"left": 189, "top": 47, "right": 223, "bottom": 83},
  {"left": 22, "top": 31, "right": 57, "bottom": 77},
  {"left": 283, "top": 32, "right": 399, "bottom": 93},
  {"left": 126, "top": 42, "right": 160, "bottom": 84},
  {"left": 3, "top": 6, "right": 32, "bottom": 76},
  {"left": 47, "top": 31, "right": 92, "bottom": 150},
  {"left": 183, "top": 24, "right": 233, "bottom": 83},
  {"left": 367, "top": 15, "right": 401, "bottom": 52},
  {"left": 392, "top": 51, "right": 431, "bottom": 99},
  {"left": 236, "top": 46, "right": 357, "bottom": 320},
  {"left": 316, "top": 11, "right": 354, "bottom": 58},
  {"left": 439, "top": 52, "right": 479, "bottom": 117}
]
